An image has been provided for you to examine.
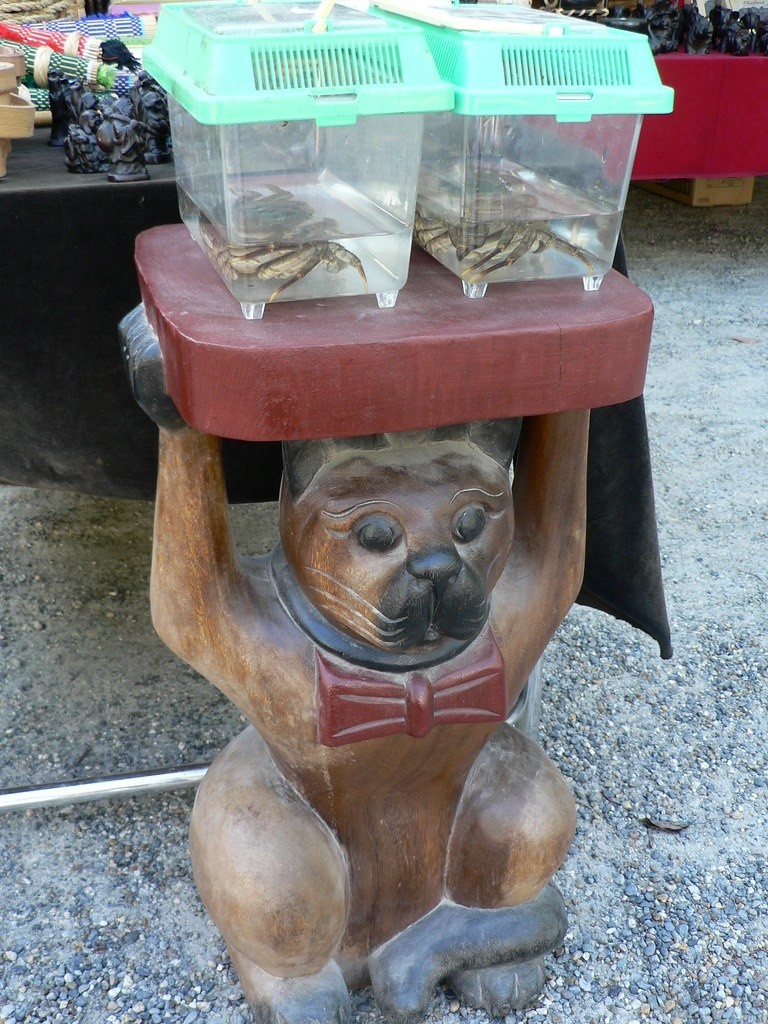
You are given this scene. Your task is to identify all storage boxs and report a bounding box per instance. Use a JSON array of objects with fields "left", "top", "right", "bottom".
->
[
  {"left": 631, "top": 176, "right": 755, "bottom": 207},
  {"left": 142, "top": 2, "right": 458, "bottom": 321},
  {"left": 368, "top": 2, "right": 675, "bottom": 298}
]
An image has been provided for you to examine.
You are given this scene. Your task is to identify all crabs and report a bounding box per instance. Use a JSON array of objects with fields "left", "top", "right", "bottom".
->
[
  {"left": 198, "top": 183, "right": 369, "bottom": 302},
  {"left": 412, "top": 164, "right": 597, "bottom": 283}
]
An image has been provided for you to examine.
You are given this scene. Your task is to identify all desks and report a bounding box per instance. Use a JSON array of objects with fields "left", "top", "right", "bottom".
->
[
  {"left": 632, "top": 52, "right": 768, "bottom": 180},
  {"left": 0, "top": 123, "right": 174, "bottom": 198}
]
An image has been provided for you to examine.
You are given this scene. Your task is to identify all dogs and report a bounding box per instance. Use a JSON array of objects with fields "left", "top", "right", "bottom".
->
[{"left": 112, "top": 303, "right": 589, "bottom": 1022}]
